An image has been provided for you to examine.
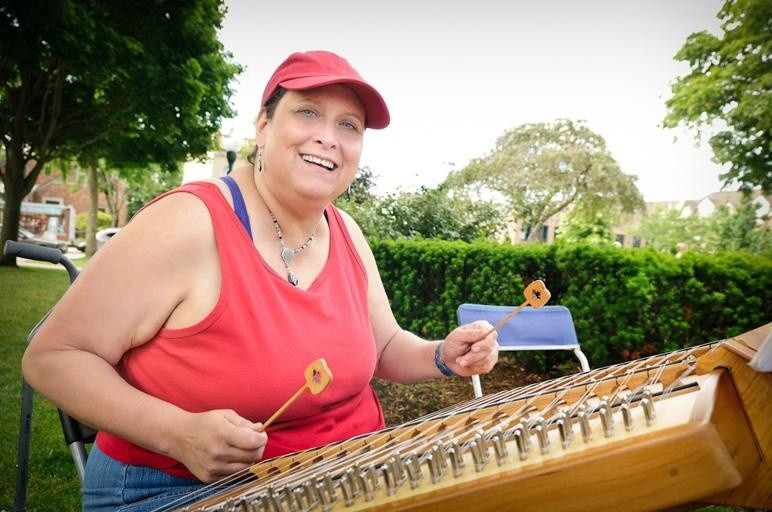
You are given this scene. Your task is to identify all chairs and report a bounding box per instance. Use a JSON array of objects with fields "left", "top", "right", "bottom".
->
[
  {"left": 456, "top": 304, "right": 591, "bottom": 399},
  {"left": 57, "top": 405, "right": 98, "bottom": 485}
]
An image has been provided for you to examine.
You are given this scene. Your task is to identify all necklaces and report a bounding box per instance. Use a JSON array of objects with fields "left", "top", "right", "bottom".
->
[{"left": 267, "top": 207, "right": 315, "bottom": 285}]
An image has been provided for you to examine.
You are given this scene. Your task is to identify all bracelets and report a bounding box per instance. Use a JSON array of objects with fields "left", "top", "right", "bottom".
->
[{"left": 435, "top": 341, "right": 455, "bottom": 378}]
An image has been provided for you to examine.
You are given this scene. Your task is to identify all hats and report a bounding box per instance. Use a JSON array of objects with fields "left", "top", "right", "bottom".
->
[{"left": 260, "top": 51, "right": 389, "bottom": 130}]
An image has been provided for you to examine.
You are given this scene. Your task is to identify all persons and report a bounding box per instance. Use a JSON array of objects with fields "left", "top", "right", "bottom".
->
[{"left": 21, "top": 51, "right": 499, "bottom": 511}]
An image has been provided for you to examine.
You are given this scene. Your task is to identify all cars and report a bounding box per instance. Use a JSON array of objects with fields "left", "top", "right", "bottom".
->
[{"left": 0, "top": 224, "right": 122, "bottom": 257}]
